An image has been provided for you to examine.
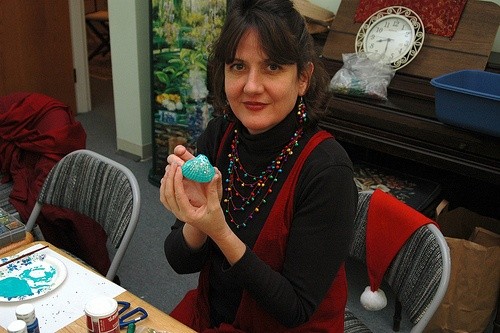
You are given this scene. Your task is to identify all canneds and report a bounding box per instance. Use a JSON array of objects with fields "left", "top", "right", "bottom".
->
[{"left": 84, "top": 297, "right": 120, "bottom": 333}]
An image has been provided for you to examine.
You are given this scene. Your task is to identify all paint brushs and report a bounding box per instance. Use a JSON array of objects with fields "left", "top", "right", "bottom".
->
[{"left": 0, "top": 246, "right": 49, "bottom": 267}]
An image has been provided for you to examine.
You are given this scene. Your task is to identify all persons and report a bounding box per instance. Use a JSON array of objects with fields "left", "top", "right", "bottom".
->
[{"left": 159, "top": 0, "right": 358, "bottom": 333}]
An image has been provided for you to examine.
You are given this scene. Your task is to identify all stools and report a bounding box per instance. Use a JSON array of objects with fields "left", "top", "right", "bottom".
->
[{"left": 344, "top": 150, "right": 450, "bottom": 332}]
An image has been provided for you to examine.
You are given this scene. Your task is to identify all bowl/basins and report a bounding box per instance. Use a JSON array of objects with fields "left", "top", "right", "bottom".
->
[{"left": 430, "top": 68, "right": 500, "bottom": 139}]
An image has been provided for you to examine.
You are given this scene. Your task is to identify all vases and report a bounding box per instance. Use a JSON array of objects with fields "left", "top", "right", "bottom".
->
[{"left": 148, "top": 113, "right": 205, "bottom": 188}]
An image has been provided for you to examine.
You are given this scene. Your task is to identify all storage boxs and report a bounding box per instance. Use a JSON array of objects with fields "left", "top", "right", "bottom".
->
[{"left": 430, "top": 68, "right": 500, "bottom": 138}]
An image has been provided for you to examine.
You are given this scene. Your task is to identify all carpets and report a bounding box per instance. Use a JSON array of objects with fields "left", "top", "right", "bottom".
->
[{"left": 86, "top": 30, "right": 112, "bottom": 81}]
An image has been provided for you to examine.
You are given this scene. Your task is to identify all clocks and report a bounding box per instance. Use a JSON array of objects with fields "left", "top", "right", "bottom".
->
[{"left": 354, "top": 6, "right": 425, "bottom": 73}]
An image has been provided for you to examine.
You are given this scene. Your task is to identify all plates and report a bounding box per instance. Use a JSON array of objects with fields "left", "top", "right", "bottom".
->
[{"left": 0, "top": 255, "right": 67, "bottom": 300}]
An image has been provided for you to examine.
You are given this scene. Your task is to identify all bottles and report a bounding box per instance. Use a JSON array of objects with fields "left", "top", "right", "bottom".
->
[
  {"left": 83, "top": 297, "right": 121, "bottom": 333},
  {"left": 8, "top": 321, "right": 28, "bottom": 333},
  {"left": 16, "top": 303, "right": 40, "bottom": 333}
]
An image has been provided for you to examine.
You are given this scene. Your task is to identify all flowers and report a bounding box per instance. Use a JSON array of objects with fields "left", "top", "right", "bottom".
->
[{"left": 151, "top": 0, "right": 227, "bottom": 124}]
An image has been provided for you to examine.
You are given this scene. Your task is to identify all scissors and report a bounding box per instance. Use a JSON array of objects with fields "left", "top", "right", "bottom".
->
[{"left": 115, "top": 301, "right": 148, "bottom": 326}]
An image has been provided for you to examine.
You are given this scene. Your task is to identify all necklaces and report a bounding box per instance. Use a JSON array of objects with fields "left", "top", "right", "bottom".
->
[{"left": 223, "top": 104, "right": 307, "bottom": 229}]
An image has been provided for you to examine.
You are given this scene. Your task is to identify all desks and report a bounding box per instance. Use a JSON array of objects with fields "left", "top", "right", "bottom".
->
[
  {"left": 312, "top": 36, "right": 500, "bottom": 189},
  {"left": 0, "top": 241, "right": 198, "bottom": 333}
]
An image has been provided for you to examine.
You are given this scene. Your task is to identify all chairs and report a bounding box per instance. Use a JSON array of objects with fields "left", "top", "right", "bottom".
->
[
  {"left": 85, "top": 0, "right": 111, "bottom": 62},
  {"left": 344, "top": 191, "right": 450, "bottom": 333},
  {"left": 0, "top": 91, "right": 87, "bottom": 242},
  {"left": 25, "top": 149, "right": 142, "bottom": 282}
]
